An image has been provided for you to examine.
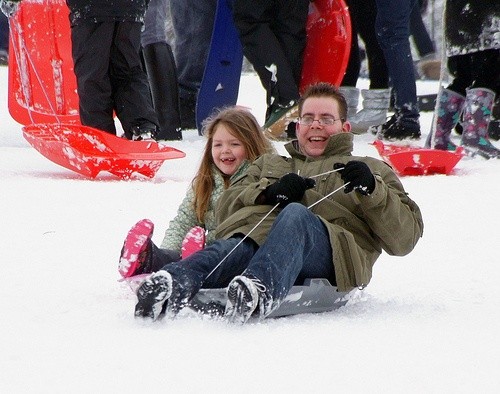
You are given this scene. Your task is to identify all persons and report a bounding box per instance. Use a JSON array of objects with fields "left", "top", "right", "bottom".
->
[
  {"left": 0, "top": 0, "right": 500, "bottom": 160},
  {"left": 117, "top": 83, "right": 424, "bottom": 325}
]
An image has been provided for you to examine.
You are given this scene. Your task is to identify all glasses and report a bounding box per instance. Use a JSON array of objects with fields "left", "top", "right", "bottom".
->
[{"left": 296, "top": 115, "right": 345, "bottom": 126}]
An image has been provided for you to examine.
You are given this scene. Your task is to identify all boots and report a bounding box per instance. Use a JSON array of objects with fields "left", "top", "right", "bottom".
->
[
  {"left": 141, "top": 42, "right": 182, "bottom": 140},
  {"left": 345, "top": 88, "right": 393, "bottom": 134},
  {"left": 459, "top": 87, "right": 500, "bottom": 161},
  {"left": 422, "top": 87, "right": 467, "bottom": 152},
  {"left": 177, "top": 85, "right": 199, "bottom": 129},
  {"left": 419, "top": 59, "right": 444, "bottom": 111},
  {"left": 337, "top": 87, "right": 361, "bottom": 123}
]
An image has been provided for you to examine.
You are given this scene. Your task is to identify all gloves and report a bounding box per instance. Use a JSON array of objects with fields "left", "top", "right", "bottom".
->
[
  {"left": 332, "top": 159, "right": 378, "bottom": 196},
  {"left": 261, "top": 173, "right": 318, "bottom": 208}
]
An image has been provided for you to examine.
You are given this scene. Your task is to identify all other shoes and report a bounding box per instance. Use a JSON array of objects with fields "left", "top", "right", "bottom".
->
[
  {"left": 259, "top": 100, "right": 303, "bottom": 140},
  {"left": 371, "top": 113, "right": 400, "bottom": 136},
  {"left": 222, "top": 275, "right": 260, "bottom": 327},
  {"left": 129, "top": 122, "right": 158, "bottom": 142},
  {"left": 117, "top": 218, "right": 156, "bottom": 277},
  {"left": 132, "top": 270, "right": 174, "bottom": 320},
  {"left": 379, "top": 116, "right": 421, "bottom": 139},
  {"left": 181, "top": 227, "right": 206, "bottom": 258}
]
